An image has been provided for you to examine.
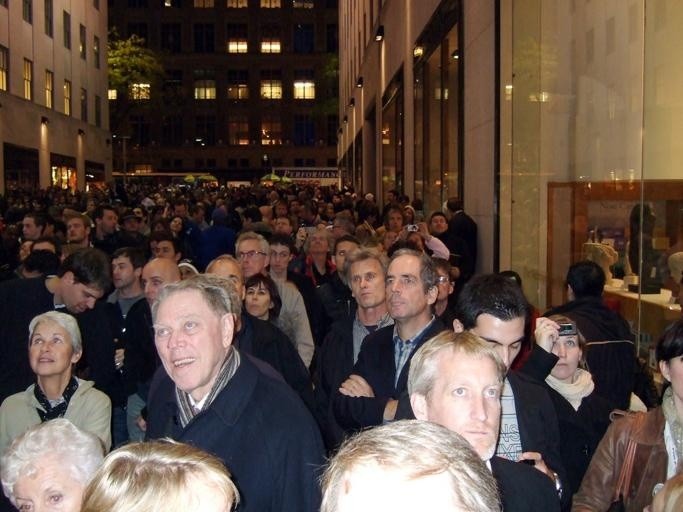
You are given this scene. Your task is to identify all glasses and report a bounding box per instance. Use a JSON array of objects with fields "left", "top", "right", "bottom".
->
[
  {"left": 436, "top": 276, "right": 449, "bottom": 285},
  {"left": 236, "top": 250, "right": 266, "bottom": 258}
]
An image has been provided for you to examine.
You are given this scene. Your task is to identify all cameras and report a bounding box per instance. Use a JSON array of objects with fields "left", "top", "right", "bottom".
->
[{"left": 407, "top": 224, "right": 418, "bottom": 232}]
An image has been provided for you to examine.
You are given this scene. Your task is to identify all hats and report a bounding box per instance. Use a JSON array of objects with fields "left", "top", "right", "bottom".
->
[{"left": 118, "top": 210, "right": 142, "bottom": 224}]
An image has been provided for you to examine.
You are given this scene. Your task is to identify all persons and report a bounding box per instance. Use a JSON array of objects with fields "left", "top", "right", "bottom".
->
[
  {"left": 79, "top": 435, "right": 242, "bottom": 512},
  {"left": 568, "top": 317, "right": 683, "bottom": 512},
  {"left": 181, "top": 186, "right": 234, "bottom": 254},
  {"left": 268, "top": 233, "right": 329, "bottom": 350},
  {"left": 0, "top": 246, "right": 114, "bottom": 407},
  {"left": 126, "top": 258, "right": 181, "bottom": 392},
  {"left": 87, "top": 180, "right": 154, "bottom": 245},
  {"left": 313, "top": 248, "right": 395, "bottom": 431},
  {"left": 521, "top": 314, "right": 621, "bottom": 499},
  {"left": 407, "top": 331, "right": 560, "bottom": 511},
  {"left": 453, "top": 272, "right": 560, "bottom": 471},
  {"left": 445, "top": 197, "right": 478, "bottom": 277},
  {"left": 642, "top": 473, "right": 683, "bottom": 512},
  {"left": 0, "top": 186, "right": 87, "bottom": 252},
  {"left": 0, "top": 416, "right": 104, "bottom": 512},
  {"left": 326, "top": 181, "right": 450, "bottom": 260},
  {"left": 128, "top": 273, "right": 328, "bottom": 512},
  {"left": 332, "top": 247, "right": 444, "bottom": 426},
  {"left": 154, "top": 185, "right": 181, "bottom": 235},
  {"left": 105, "top": 246, "right": 153, "bottom": 405},
  {"left": 522, "top": 259, "right": 658, "bottom": 412},
  {"left": 148, "top": 254, "right": 310, "bottom": 392},
  {"left": 155, "top": 235, "right": 181, "bottom": 263},
  {"left": 314, "top": 235, "right": 358, "bottom": 335},
  {"left": 235, "top": 232, "right": 316, "bottom": 370},
  {"left": 317, "top": 420, "right": 504, "bottom": 512},
  {"left": 245, "top": 274, "right": 296, "bottom": 350},
  {"left": 1, "top": 249, "right": 61, "bottom": 281},
  {"left": 178, "top": 258, "right": 200, "bottom": 282},
  {"left": 432, "top": 256, "right": 456, "bottom": 331},
  {"left": 0, "top": 311, "right": 112, "bottom": 456},
  {"left": 234, "top": 180, "right": 326, "bottom": 232}
]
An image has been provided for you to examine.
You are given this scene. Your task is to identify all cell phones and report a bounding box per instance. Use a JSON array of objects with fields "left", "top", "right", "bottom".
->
[
  {"left": 513, "top": 458, "right": 536, "bottom": 467},
  {"left": 556, "top": 321, "right": 577, "bottom": 336}
]
